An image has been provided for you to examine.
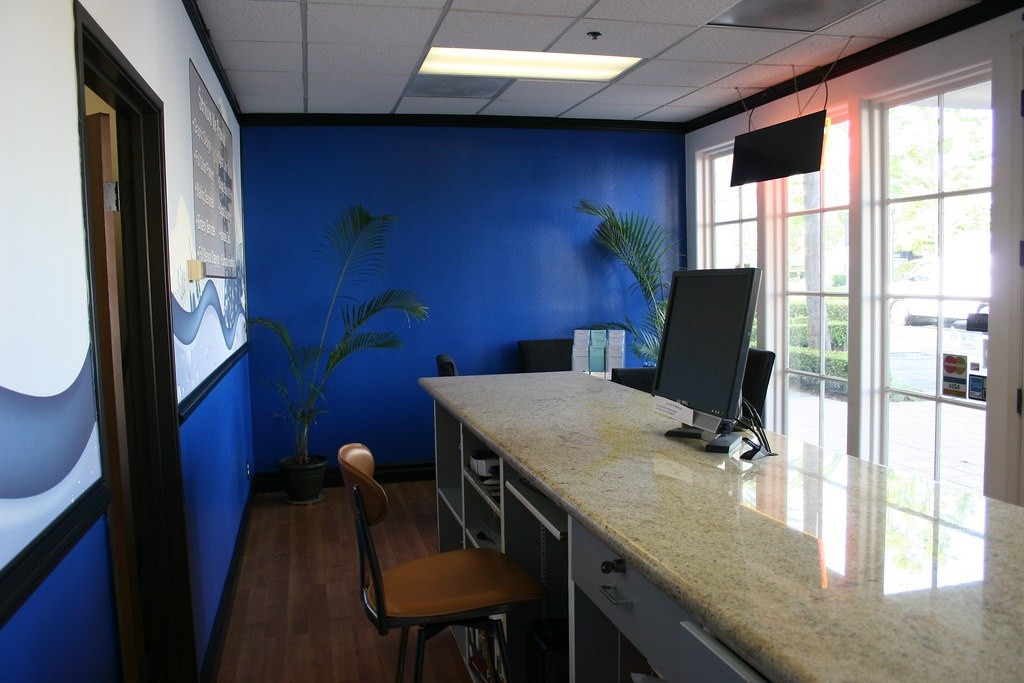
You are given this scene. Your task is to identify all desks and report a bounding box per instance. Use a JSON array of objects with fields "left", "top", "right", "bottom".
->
[{"left": 419, "top": 368, "right": 1024, "bottom": 681}]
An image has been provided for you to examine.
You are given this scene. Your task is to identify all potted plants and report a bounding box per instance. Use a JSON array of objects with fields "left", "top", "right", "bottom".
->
[{"left": 244, "top": 192, "right": 431, "bottom": 505}]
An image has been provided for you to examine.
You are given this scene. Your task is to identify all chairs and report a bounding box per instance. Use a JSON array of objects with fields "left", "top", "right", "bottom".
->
[
  {"left": 611, "top": 364, "right": 659, "bottom": 393},
  {"left": 736, "top": 349, "right": 777, "bottom": 427},
  {"left": 337, "top": 436, "right": 548, "bottom": 678},
  {"left": 517, "top": 338, "right": 581, "bottom": 373},
  {"left": 434, "top": 353, "right": 460, "bottom": 377}
]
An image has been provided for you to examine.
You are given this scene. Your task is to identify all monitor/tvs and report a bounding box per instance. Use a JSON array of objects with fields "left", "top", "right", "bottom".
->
[{"left": 649, "top": 266, "right": 764, "bottom": 423}]
